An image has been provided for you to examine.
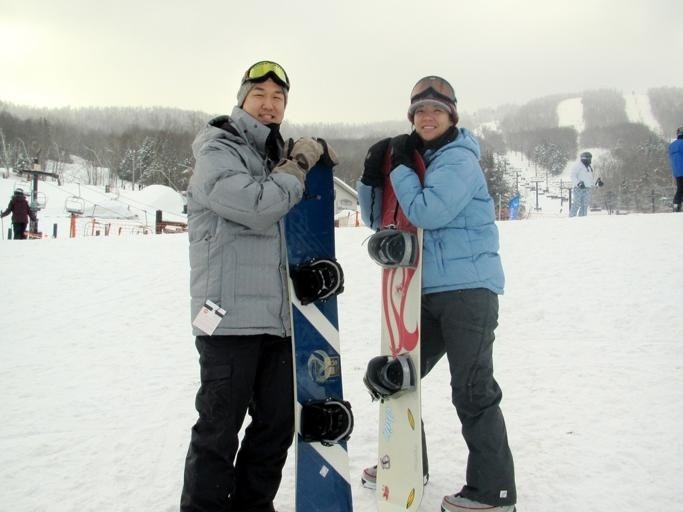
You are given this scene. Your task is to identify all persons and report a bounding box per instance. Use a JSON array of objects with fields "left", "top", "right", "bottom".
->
[
  {"left": 177, "top": 59, "right": 338, "bottom": 511},
  {"left": 0, "top": 187, "right": 36, "bottom": 241},
  {"left": 564, "top": 152, "right": 603, "bottom": 216},
  {"left": 668, "top": 127, "right": 682, "bottom": 211},
  {"left": 358, "top": 75, "right": 524, "bottom": 510}
]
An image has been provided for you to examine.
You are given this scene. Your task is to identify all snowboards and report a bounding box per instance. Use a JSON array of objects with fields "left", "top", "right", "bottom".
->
[
  {"left": 364, "top": 139, "right": 423, "bottom": 512},
  {"left": 277, "top": 136, "right": 354, "bottom": 511}
]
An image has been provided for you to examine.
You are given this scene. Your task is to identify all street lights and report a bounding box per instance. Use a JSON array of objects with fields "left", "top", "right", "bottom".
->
[{"left": 495, "top": 193, "right": 501, "bottom": 221}]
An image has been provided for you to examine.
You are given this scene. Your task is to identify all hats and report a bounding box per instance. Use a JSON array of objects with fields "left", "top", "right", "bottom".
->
[
  {"left": 407, "top": 95, "right": 458, "bottom": 126},
  {"left": 237, "top": 76, "right": 287, "bottom": 110},
  {"left": 579, "top": 151, "right": 592, "bottom": 160},
  {"left": 13, "top": 188, "right": 24, "bottom": 194},
  {"left": 675, "top": 127, "right": 683, "bottom": 134}
]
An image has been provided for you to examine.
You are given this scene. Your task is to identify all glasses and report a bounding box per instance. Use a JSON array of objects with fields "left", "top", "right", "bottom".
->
[
  {"left": 241, "top": 61, "right": 290, "bottom": 92},
  {"left": 410, "top": 75, "right": 457, "bottom": 103}
]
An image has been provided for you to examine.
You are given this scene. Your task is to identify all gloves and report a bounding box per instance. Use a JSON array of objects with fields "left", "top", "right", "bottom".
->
[
  {"left": 273, "top": 134, "right": 324, "bottom": 180},
  {"left": 391, "top": 133, "right": 418, "bottom": 172},
  {"left": 360, "top": 136, "right": 390, "bottom": 186},
  {"left": 316, "top": 136, "right": 340, "bottom": 166}
]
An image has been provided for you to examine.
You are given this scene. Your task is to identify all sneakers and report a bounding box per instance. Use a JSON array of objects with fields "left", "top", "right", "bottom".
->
[
  {"left": 362, "top": 463, "right": 430, "bottom": 491},
  {"left": 441, "top": 490, "right": 518, "bottom": 512},
  {"left": 673, "top": 204, "right": 683, "bottom": 211}
]
